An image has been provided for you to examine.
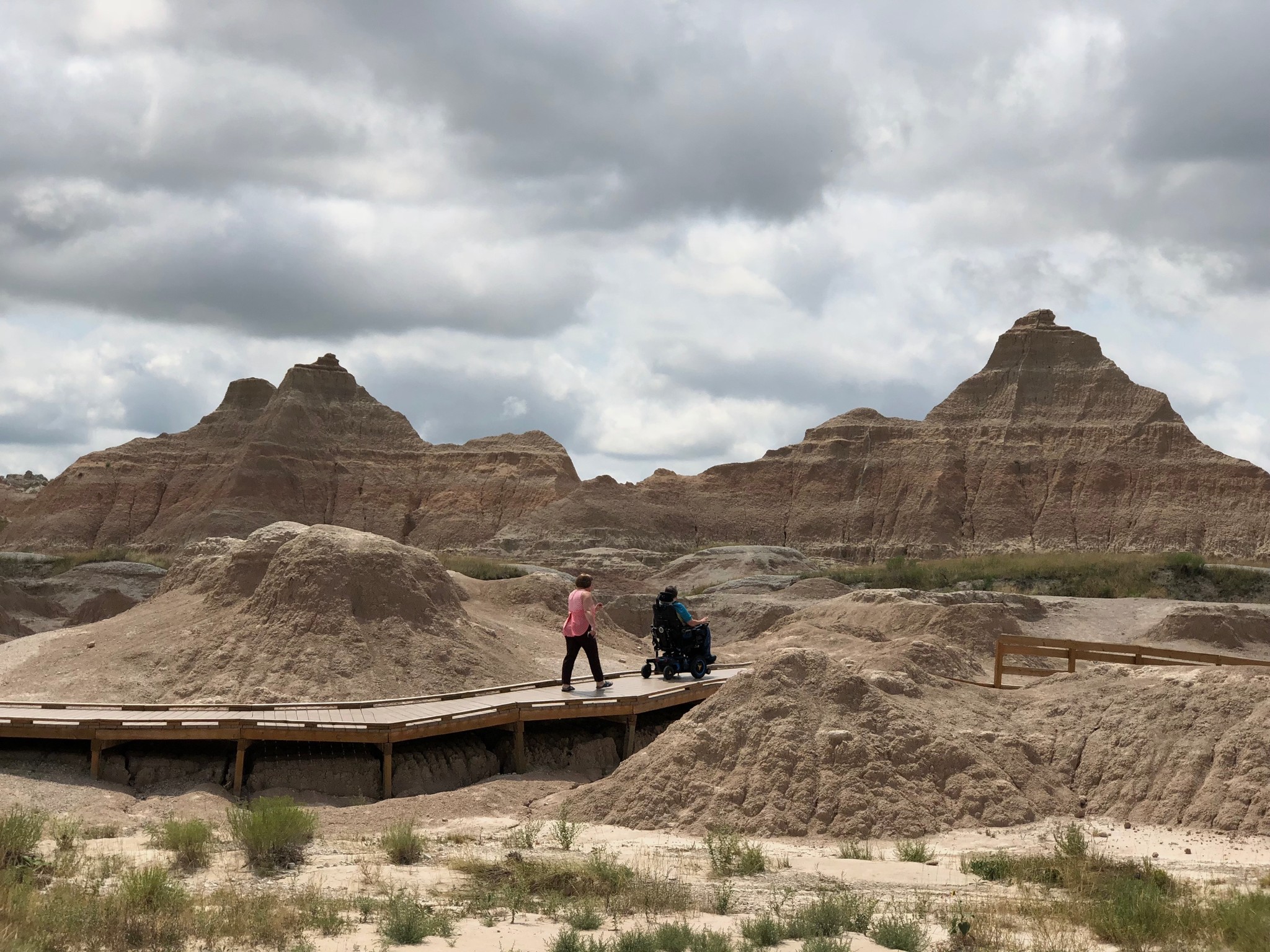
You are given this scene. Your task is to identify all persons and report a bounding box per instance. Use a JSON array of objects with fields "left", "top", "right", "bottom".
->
[
  {"left": 659, "top": 585, "right": 716, "bottom": 665},
  {"left": 561, "top": 573, "right": 614, "bottom": 692}
]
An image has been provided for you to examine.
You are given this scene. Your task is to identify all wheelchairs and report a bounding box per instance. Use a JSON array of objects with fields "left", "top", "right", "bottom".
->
[{"left": 641, "top": 592, "right": 716, "bottom": 680}]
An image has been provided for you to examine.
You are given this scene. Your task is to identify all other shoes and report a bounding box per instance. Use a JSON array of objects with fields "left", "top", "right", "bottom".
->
[{"left": 706, "top": 655, "right": 717, "bottom": 664}]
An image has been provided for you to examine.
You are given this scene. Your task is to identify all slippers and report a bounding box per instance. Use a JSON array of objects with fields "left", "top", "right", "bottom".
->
[
  {"left": 596, "top": 681, "right": 613, "bottom": 689},
  {"left": 561, "top": 686, "right": 575, "bottom": 692}
]
